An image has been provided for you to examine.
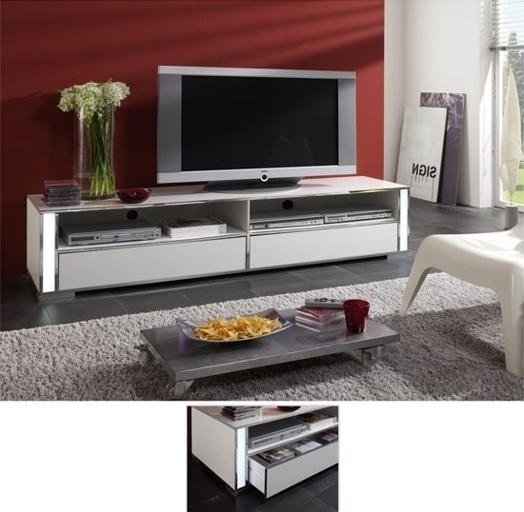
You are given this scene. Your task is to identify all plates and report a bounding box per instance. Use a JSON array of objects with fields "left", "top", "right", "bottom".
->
[{"left": 175, "top": 307, "right": 294, "bottom": 346}]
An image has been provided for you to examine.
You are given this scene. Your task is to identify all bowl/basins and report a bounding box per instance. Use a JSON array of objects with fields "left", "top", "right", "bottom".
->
[{"left": 116, "top": 188, "right": 151, "bottom": 204}]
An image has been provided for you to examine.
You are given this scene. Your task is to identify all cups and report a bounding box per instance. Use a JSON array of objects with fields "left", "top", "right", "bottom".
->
[{"left": 342, "top": 298, "right": 370, "bottom": 334}]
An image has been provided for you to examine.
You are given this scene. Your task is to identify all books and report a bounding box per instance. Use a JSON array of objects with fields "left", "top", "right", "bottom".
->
[
  {"left": 220, "top": 406, "right": 338, "bottom": 464},
  {"left": 40, "top": 178, "right": 81, "bottom": 207},
  {"left": 293, "top": 306, "right": 347, "bottom": 332}
]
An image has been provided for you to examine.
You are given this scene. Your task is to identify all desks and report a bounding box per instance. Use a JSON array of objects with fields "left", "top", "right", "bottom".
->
[{"left": 191, "top": 406, "right": 339, "bottom": 499}]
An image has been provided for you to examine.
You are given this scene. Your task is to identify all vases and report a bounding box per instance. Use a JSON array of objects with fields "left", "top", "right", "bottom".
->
[{"left": 72, "top": 108, "right": 117, "bottom": 201}]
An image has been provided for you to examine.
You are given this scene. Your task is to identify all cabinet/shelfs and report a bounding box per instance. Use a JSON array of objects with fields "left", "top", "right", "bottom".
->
[{"left": 26, "top": 175, "right": 409, "bottom": 305}]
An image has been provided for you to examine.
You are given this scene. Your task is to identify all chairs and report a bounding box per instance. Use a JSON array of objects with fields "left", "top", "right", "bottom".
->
[{"left": 398, "top": 217, "right": 524, "bottom": 378}]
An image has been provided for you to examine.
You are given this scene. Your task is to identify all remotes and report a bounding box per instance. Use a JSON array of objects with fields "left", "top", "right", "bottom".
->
[{"left": 303, "top": 297, "right": 345, "bottom": 310}]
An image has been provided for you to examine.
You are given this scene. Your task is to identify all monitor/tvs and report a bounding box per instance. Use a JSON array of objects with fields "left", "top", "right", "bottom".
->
[{"left": 157, "top": 64, "right": 356, "bottom": 193}]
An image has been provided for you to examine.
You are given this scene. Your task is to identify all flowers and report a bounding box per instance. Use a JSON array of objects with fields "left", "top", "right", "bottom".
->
[{"left": 55, "top": 77, "right": 131, "bottom": 197}]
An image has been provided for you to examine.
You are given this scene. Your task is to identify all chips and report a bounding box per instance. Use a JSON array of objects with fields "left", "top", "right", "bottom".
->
[{"left": 195, "top": 316, "right": 282, "bottom": 341}]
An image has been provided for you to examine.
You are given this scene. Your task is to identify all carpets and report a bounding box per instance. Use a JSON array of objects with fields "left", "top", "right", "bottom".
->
[{"left": 0, "top": 270, "right": 524, "bottom": 400}]
